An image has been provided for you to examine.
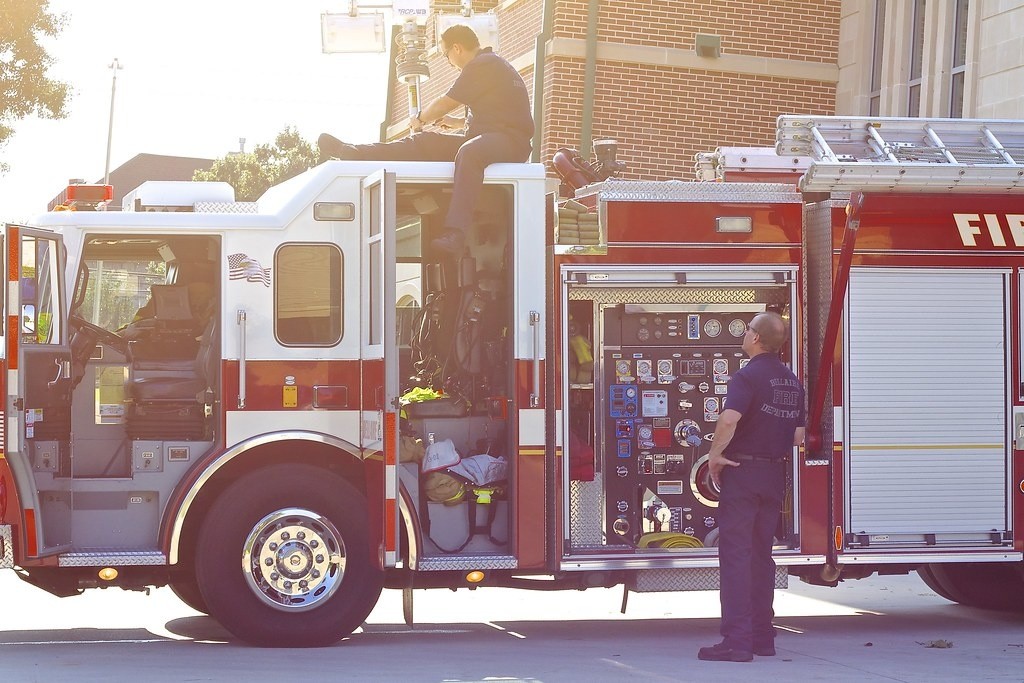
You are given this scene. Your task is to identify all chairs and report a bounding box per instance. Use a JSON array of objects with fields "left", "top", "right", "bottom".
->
[
  {"left": 130, "top": 299, "right": 219, "bottom": 441},
  {"left": 403, "top": 255, "right": 484, "bottom": 416}
]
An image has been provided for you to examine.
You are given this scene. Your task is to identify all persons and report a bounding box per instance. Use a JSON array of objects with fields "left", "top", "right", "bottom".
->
[
  {"left": 698, "top": 311, "right": 805, "bottom": 662},
  {"left": 318, "top": 25, "right": 536, "bottom": 256}
]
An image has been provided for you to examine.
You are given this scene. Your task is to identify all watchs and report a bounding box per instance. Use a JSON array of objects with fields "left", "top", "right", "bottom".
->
[{"left": 416, "top": 110, "right": 426, "bottom": 124}]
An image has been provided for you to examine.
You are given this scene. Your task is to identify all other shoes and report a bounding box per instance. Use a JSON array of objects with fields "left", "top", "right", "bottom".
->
[
  {"left": 753, "top": 645, "right": 775, "bottom": 656},
  {"left": 318, "top": 133, "right": 358, "bottom": 159},
  {"left": 431, "top": 229, "right": 465, "bottom": 255},
  {"left": 698, "top": 643, "right": 754, "bottom": 662}
]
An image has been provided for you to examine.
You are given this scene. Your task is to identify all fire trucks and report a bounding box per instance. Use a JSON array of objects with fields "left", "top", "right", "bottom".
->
[{"left": 0, "top": 117, "right": 1022, "bottom": 648}]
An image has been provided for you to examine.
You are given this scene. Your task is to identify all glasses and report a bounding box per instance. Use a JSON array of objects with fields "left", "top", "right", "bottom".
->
[
  {"left": 443, "top": 46, "right": 454, "bottom": 60},
  {"left": 747, "top": 323, "right": 761, "bottom": 336}
]
